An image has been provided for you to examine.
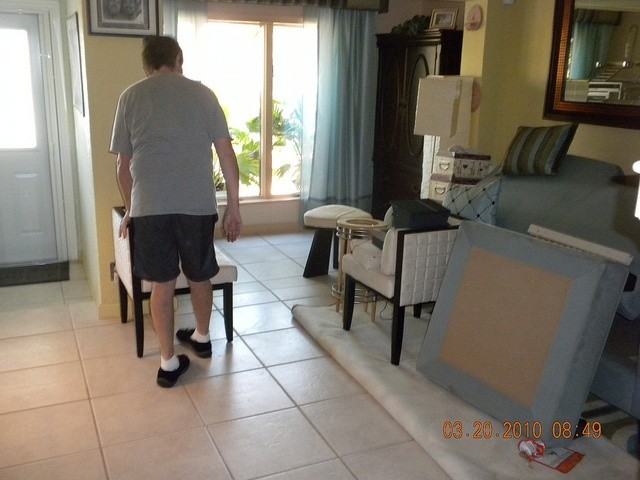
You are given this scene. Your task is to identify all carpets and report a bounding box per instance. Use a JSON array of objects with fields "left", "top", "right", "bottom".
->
[{"left": 291, "top": 305, "right": 640, "bottom": 480}]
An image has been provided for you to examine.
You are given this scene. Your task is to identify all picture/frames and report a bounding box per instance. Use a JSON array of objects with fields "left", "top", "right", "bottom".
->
[
  {"left": 85, "top": 0, "right": 164, "bottom": 40},
  {"left": 429, "top": 6, "right": 464, "bottom": 32}
]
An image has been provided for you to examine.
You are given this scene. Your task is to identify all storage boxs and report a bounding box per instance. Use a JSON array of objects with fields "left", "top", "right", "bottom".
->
[
  {"left": 427, "top": 152, "right": 491, "bottom": 181},
  {"left": 426, "top": 171, "right": 480, "bottom": 214}
]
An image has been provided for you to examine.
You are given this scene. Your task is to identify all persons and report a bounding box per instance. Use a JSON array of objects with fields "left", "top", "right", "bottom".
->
[{"left": 108, "top": 34, "right": 243, "bottom": 388}]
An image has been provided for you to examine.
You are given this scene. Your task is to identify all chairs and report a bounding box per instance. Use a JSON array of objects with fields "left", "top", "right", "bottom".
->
[
  {"left": 341, "top": 227, "right": 463, "bottom": 366},
  {"left": 110, "top": 204, "right": 239, "bottom": 358}
]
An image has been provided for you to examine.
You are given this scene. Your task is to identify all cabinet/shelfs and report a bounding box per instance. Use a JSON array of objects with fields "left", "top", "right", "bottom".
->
[{"left": 371, "top": 15, "right": 465, "bottom": 221}]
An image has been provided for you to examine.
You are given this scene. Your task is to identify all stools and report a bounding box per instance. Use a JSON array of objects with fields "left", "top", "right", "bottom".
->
[
  {"left": 304, "top": 204, "right": 372, "bottom": 278},
  {"left": 329, "top": 216, "right": 389, "bottom": 314}
]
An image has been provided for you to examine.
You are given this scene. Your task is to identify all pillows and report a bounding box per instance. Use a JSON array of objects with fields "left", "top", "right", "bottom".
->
[{"left": 502, "top": 124, "right": 578, "bottom": 174}]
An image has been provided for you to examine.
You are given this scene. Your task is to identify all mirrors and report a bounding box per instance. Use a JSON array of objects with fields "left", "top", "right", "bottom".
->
[{"left": 541, "top": 0, "right": 639, "bottom": 130}]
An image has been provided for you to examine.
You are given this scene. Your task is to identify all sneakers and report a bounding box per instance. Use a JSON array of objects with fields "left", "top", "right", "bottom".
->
[
  {"left": 157, "top": 354, "right": 190, "bottom": 388},
  {"left": 175, "top": 328, "right": 212, "bottom": 358}
]
